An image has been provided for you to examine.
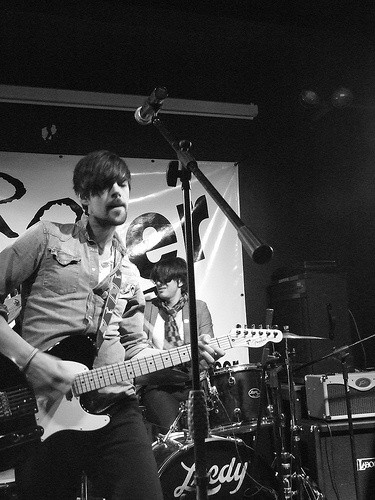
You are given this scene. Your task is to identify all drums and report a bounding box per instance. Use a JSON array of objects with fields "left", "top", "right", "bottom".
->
[
  {"left": 198, "top": 359, "right": 274, "bottom": 439},
  {"left": 151, "top": 433, "right": 283, "bottom": 500}
]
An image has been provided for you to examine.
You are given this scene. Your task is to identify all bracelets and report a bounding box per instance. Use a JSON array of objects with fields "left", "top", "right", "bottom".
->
[{"left": 19, "top": 348, "right": 39, "bottom": 371}]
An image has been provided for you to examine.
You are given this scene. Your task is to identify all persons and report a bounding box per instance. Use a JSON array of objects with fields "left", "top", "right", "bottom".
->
[
  {"left": 135, "top": 257, "right": 214, "bottom": 435},
  {"left": 0, "top": 150, "right": 226, "bottom": 500}
]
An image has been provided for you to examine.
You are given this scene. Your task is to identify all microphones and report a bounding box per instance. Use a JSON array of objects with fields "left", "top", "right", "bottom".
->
[{"left": 135, "top": 86, "right": 168, "bottom": 126}]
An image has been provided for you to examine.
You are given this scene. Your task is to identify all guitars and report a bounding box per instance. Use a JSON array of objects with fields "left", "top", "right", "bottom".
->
[{"left": 0, "top": 323, "right": 285, "bottom": 453}]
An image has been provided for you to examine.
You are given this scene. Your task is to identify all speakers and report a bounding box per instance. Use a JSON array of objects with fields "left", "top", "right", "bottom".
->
[
  {"left": 296, "top": 419, "right": 375, "bottom": 499},
  {"left": 266, "top": 270, "right": 354, "bottom": 378}
]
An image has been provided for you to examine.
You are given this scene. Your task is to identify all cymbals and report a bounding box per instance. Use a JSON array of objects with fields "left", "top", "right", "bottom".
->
[{"left": 283, "top": 330, "right": 328, "bottom": 342}]
